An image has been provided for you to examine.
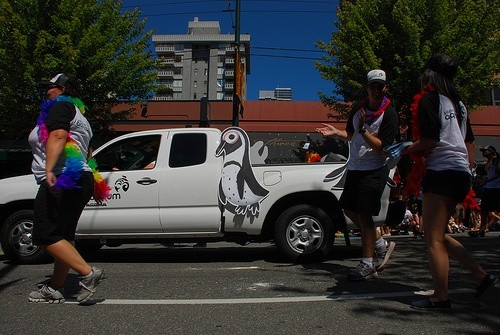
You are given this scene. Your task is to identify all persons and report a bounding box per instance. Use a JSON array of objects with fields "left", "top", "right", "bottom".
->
[
  {"left": 111, "top": 143, "right": 160, "bottom": 172},
  {"left": 395, "top": 54, "right": 500, "bottom": 308},
  {"left": 292, "top": 131, "right": 366, "bottom": 246},
  {"left": 193, "top": 242, "right": 207, "bottom": 248},
  {"left": 25, "top": 73, "right": 106, "bottom": 305},
  {"left": 314, "top": 67, "right": 398, "bottom": 274},
  {"left": 158, "top": 241, "right": 175, "bottom": 247},
  {"left": 388, "top": 122, "right": 500, "bottom": 239}
]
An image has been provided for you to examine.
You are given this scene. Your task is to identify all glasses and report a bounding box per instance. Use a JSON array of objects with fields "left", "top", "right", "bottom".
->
[{"left": 46, "top": 85, "right": 59, "bottom": 91}]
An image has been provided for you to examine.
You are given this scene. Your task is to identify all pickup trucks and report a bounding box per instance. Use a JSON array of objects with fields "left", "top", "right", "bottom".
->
[{"left": 0, "top": 128, "right": 406, "bottom": 266}]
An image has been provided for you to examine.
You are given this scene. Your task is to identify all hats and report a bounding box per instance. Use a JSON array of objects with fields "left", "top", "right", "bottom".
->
[
  {"left": 480, "top": 145, "right": 496, "bottom": 154},
  {"left": 38, "top": 73, "right": 78, "bottom": 90},
  {"left": 366, "top": 69, "right": 387, "bottom": 85},
  {"left": 298, "top": 142, "right": 314, "bottom": 150},
  {"left": 425, "top": 53, "right": 456, "bottom": 77}
]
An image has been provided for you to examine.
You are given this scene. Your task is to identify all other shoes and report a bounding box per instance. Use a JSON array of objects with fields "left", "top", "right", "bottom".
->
[
  {"left": 411, "top": 299, "right": 449, "bottom": 310},
  {"left": 468, "top": 230, "right": 485, "bottom": 236},
  {"left": 475, "top": 273, "right": 497, "bottom": 297}
]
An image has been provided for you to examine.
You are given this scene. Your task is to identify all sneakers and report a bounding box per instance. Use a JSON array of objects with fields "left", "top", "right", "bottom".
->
[
  {"left": 347, "top": 259, "right": 378, "bottom": 281},
  {"left": 374, "top": 240, "right": 395, "bottom": 271},
  {"left": 28, "top": 283, "right": 65, "bottom": 303},
  {"left": 77, "top": 266, "right": 105, "bottom": 303}
]
